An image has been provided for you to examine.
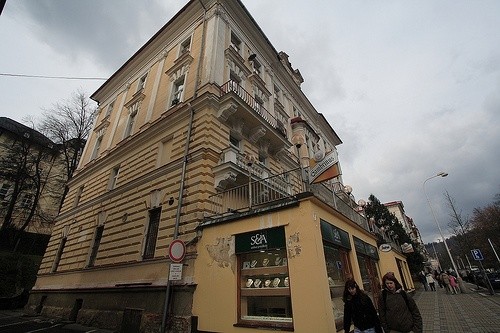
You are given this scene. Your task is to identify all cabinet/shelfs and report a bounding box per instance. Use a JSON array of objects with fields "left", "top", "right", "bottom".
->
[{"left": 240, "top": 265, "right": 290, "bottom": 297}]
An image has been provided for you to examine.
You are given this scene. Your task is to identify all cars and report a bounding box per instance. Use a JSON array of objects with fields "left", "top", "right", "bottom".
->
[{"left": 464, "top": 265, "right": 500, "bottom": 292}]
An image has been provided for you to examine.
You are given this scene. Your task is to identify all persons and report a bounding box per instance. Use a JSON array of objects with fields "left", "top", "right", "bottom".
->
[
  {"left": 343, "top": 279, "right": 381, "bottom": 333},
  {"left": 419, "top": 269, "right": 460, "bottom": 295},
  {"left": 375, "top": 272, "right": 422, "bottom": 333}
]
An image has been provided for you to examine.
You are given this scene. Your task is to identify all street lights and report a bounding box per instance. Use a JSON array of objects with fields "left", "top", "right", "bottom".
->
[{"left": 423, "top": 171, "right": 467, "bottom": 294}]
